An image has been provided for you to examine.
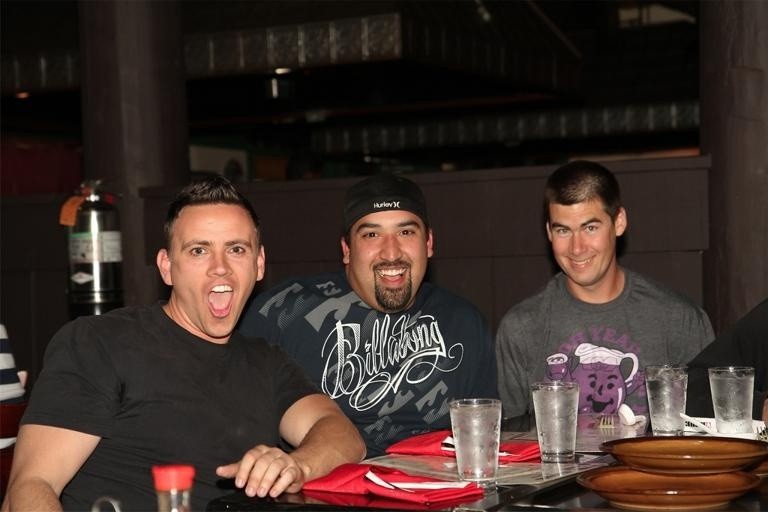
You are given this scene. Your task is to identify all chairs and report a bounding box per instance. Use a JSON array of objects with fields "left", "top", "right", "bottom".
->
[{"left": 0, "top": 400, "right": 28, "bottom": 504}]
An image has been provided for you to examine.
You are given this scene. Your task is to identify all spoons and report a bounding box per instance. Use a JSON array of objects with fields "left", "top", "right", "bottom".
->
[
  {"left": 617, "top": 402, "right": 646, "bottom": 427},
  {"left": 676, "top": 412, "right": 756, "bottom": 438}
]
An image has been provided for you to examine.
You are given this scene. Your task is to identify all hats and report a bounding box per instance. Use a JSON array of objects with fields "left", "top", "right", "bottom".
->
[{"left": 341, "top": 174, "right": 432, "bottom": 232}]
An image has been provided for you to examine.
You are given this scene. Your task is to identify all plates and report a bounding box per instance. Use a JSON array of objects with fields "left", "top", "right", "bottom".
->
[
  {"left": 598, "top": 434, "right": 765, "bottom": 473},
  {"left": 576, "top": 465, "right": 764, "bottom": 507}
]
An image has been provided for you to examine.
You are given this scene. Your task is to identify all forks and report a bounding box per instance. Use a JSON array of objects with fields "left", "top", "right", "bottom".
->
[{"left": 587, "top": 405, "right": 616, "bottom": 432}]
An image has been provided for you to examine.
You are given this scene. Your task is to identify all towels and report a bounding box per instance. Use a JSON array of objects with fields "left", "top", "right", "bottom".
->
[
  {"left": 302, "top": 462, "right": 485, "bottom": 506},
  {"left": 385, "top": 430, "right": 541, "bottom": 464}
]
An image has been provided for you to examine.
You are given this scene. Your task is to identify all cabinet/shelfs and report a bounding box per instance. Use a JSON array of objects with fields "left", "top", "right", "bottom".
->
[{"left": 2, "top": 0, "right": 715, "bottom": 399}]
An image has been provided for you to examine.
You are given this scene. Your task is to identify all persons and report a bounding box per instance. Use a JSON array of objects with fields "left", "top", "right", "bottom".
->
[
  {"left": 1, "top": 175, "right": 366, "bottom": 511},
  {"left": 238, "top": 178, "right": 498, "bottom": 457},
  {"left": 494, "top": 162, "right": 714, "bottom": 429},
  {"left": 669, "top": 292, "right": 768, "bottom": 422}
]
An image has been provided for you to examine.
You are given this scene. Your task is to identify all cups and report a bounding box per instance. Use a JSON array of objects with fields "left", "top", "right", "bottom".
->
[
  {"left": 644, "top": 366, "right": 690, "bottom": 438},
  {"left": 449, "top": 399, "right": 502, "bottom": 482},
  {"left": 533, "top": 382, "right": 581, "bottom": 463},
  {"left": 707, "top": 368, "right": 756, "bottom": 434}
]
